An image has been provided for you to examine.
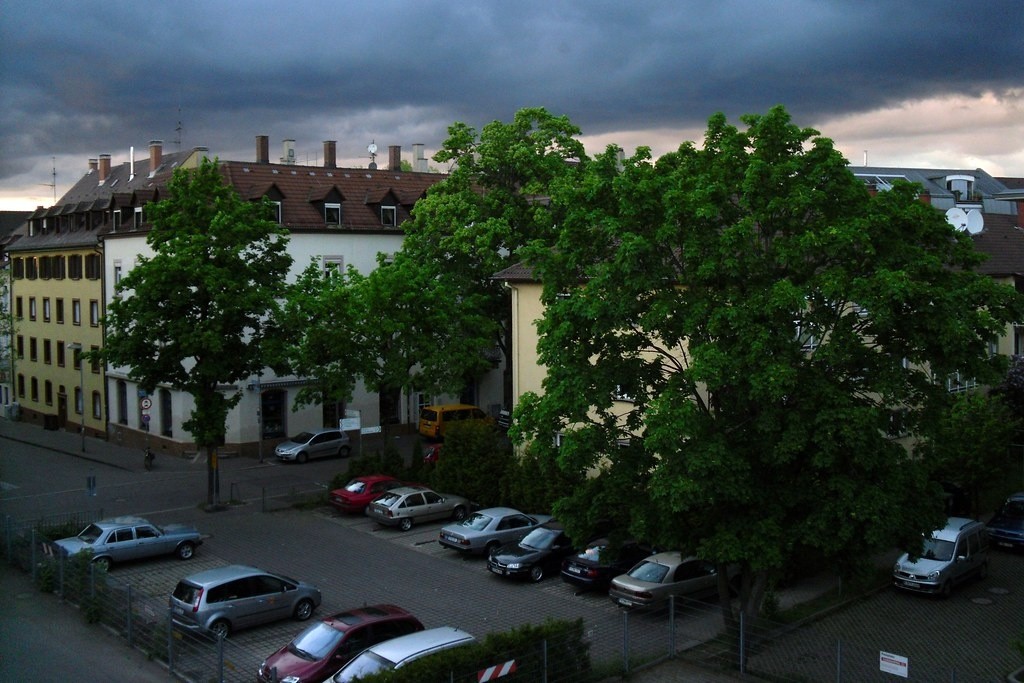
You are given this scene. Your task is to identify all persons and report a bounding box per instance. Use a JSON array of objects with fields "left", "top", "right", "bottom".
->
[{"left": 143, "top": 446, "right": 154, "bottom": 471}]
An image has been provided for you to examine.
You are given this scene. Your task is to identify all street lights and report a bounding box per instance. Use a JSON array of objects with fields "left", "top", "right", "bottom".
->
[{"left": 67, "top": 343, "right": 84, "bottom": 452}]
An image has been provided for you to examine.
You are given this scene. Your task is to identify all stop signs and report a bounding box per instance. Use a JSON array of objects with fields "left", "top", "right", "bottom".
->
[
  {"left": 141, "top": 397, "right": 152, "bottom": 410},
  {"left": 143, "top": 415, "right": 150, "bottom": 422}
]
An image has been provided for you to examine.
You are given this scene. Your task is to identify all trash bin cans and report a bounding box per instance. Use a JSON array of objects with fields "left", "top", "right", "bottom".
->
[
  {"left": 43, "top": 414, "right": 59, "bottom": 430},
  {"left": 11, "top": 402, "right": 20, "bottom": 421},
  {"left": 3, "top": 405, "right": 12, "bottom": 420}
]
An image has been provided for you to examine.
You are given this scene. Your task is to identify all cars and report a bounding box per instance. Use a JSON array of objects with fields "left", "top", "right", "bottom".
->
[
  {"left": 368, "top": 484, "right": 472, "bottom": 532},
  {"left": 424, "top": 443, "right": 444, "bottom": 466},
  {"left": 439, "top": 507, "right": 563, "bottom": 554},
  {"left": 51, "top": 516, "right": 205, "bottom": 574},
  {"left": 560, "top": 536, "right": 668, "bottom": 595},
  {"left": 487, "top": 522, "right": 574, "bottom": 581},
  {"left": 608, "top": 549, "right": 726, "bottom": 614},
  {"left": 329, "top": 473, "right": 427, "bottom": 512},
  {"left": 984, "top": 492, "right": 1024, "bottom": 552}
]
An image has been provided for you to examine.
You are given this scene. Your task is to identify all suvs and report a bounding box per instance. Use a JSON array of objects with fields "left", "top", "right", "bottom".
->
[
  {"left": 322, "top": 626, "right": 480, "bottom": 683},
  {"left": 256, "top": 603, "right": 425, "bottom": 683},
  {"left": 890, "top": 514, "right": 988, "bottom": 599},
  {"left": 275, "top": 427, "right": 352, "bottom": 463},
  {"left": 168, "top": 563, "right": 322, "bottom": 642}
]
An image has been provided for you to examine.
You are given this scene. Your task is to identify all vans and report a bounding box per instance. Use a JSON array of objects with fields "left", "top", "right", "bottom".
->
[
  {"left": 498, "top": 400, "right": 510, "bottom": 428},
  {"left": 418, "top": 403, "right": 497, "bottom": 439}
]
